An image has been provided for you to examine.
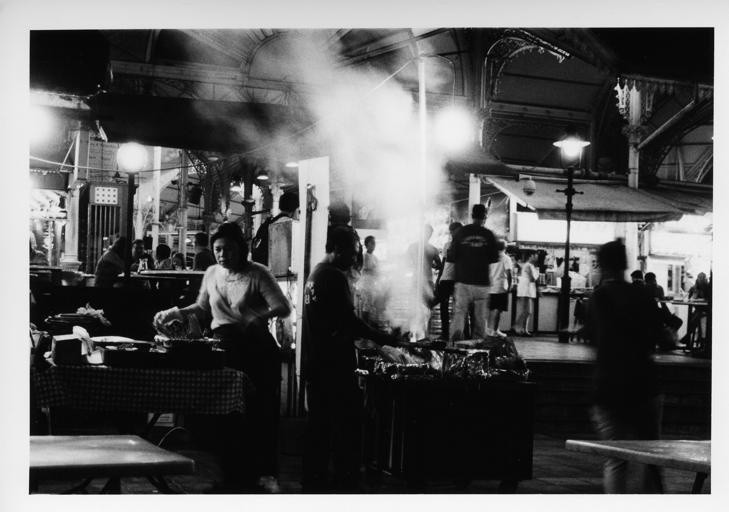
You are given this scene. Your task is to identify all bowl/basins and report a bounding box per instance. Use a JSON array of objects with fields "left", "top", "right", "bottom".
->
[
  {"left": 61, "top": 261, "right": 83, "bottom": 273},
  {"left": 177, "top": 343, "right": 226, "bottom": 371}
]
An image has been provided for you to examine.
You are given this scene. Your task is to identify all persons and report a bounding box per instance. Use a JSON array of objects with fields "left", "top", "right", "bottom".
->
[{"left": 31, "top": 184, "right": 712, "bottom": 495}]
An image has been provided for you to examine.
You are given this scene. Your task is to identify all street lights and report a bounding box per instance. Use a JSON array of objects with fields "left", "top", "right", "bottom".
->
[
  {"left": 110, "top": 140, "right": 150, "bottom": 278},
  {"left": 548, "top": 130, "right": 595, "bottom": 342}
]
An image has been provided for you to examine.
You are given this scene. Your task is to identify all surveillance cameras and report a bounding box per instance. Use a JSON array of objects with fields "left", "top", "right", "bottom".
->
[{"left": 523, "top": 180, "right": 536, "bottom": 195}]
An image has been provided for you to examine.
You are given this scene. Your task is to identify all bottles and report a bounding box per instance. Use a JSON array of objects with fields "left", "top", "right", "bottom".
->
[{"left": 137, "top": 258, "right": 148, "bottom": 274}]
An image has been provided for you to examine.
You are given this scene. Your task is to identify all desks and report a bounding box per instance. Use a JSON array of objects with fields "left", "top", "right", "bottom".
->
[
  {"left": 657, "top": 296, "right": 708, "bottom": 354},
  {"left": 29, "top": 435, "right": 195, "bottom": 495},
  {"left": 30, "top": 351, "right": 249, "bottom": 436},
  {"left": 81, "top": 270, "right": 205, "bottom": 280},
  {"left": 566, "top": 439, "right": 711, "bottom": 494}
]
